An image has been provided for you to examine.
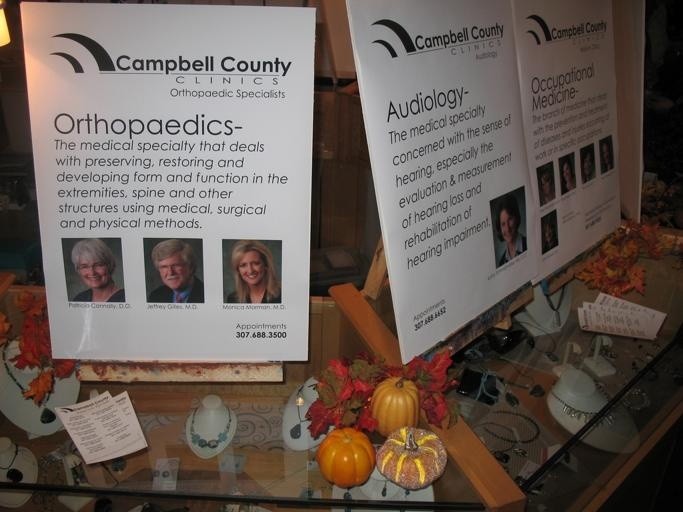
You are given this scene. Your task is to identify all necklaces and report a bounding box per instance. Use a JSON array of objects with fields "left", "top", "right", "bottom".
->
[
  {"left": 289, "top": 385, "right": 312, "bottom": 441},
  {"left": 2, "top": 339, "right": 58, "bottom": 425},
  {"left": 189, "top": 403, "right": 232, "bottom": 448},
  {"left": 1, "top": 442, "right": 25, "bottom": 483},
  {"left": 455, "top": 319, "right": 559, "bottom": 465},
  {"left": 550, "top": 376, "right": 617, "bottom": 428},
  {"left": 542, "top": 285, "right": 566, "bottom": 328}
]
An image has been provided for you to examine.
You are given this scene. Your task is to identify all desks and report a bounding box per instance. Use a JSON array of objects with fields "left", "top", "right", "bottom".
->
[
  {"left": 329, "top": 227, "right": 682, "bottom": 511},
  {"left": 0, "top": 281, "right": 494, "bottom": 510}
]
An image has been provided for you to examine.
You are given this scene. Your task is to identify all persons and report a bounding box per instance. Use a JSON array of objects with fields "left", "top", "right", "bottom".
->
[
  {"left": 70, "top": 236, "right": 125, "bottom": 305},
  {"left": 561, "top": 159, "right": 577, "bottom": 194},
  {"left": 582, "top": 150, "right": 595, "bottom": 184},
  {"left": 540, "top": 169, "right": 555, "bottom": 204},
  {"left": 494, "top": 195, "right": 527, "bottom": 265},
  {"left": 222, "top": 239, "right": 281, "bottom": 302},
  {"left": 543, "top": 220, "right": 558, "bottom": 251},
  {"left": 145, "top": 238, "right": 205, "bottom": 302},
  {"left": 602, "top": 143, "right": 614, "bottom": 171}
]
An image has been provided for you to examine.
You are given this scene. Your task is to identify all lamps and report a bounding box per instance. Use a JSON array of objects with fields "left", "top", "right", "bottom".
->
[{"left": 0, "top": 9, "right": 11, "bottom": 48}]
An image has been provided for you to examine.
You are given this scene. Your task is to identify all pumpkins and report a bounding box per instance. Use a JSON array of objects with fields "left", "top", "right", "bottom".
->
[
  {"left": 376, "top": 427, "right": 447, "bottom": 490},
  {"left": 371, "top": 377, "right": 420, "bottom": 438},
  {"left": 318, "top": 429, "right": 378, "bottom": 488}
]
